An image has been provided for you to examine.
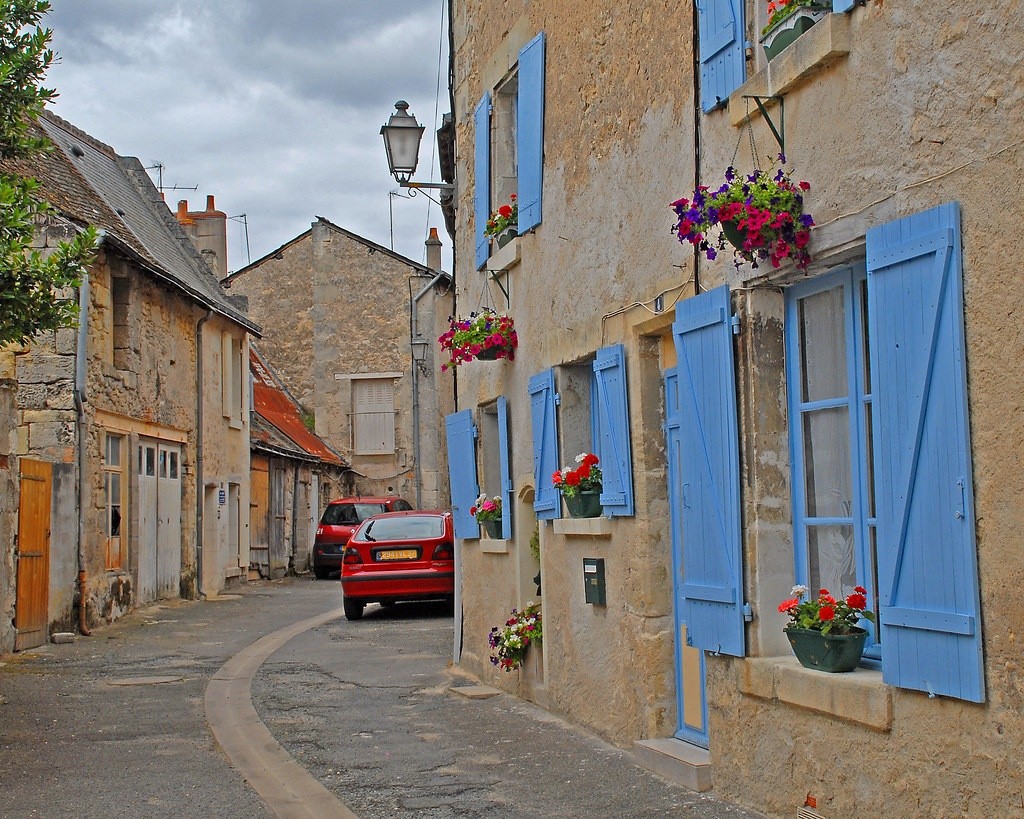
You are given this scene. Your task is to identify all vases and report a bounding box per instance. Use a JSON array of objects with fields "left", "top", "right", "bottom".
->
[
  {"left": 562, "top": 490, "right": 603, "bottom": 518},
  {"left": 493, "top": 223, "right": 518, "bottom": 249},
  {"left": 476, "top": 345, "right": 499, "bottom": 360},
  {"left": 721, "top": 219, "right": 755, "bottom": 252},
  {"left": 782, "top": 622, "right": 870, "bottom": 672},
  {"left": 481, "top": 519, "right": 502, "bottom": 539},
  {"left": 759, "top": 6, "right": 834, "bottom": 59}
]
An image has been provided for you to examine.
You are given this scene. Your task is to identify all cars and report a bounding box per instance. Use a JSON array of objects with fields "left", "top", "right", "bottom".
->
[
  {"left": 340, "top": 511, "right": 456, "bottom": 622},
  {"left": 312, "top": 496, "right": 416, "bottom": 579}
]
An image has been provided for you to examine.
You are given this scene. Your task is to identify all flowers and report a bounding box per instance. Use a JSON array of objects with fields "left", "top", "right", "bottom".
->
[
  {"left": 437, "top": 307, "right": 518, "bottom": 372},
  {"left": 470, "top": 492, "right": 502, "bottom": 522},
  {"left": 552, "top": 453, "right": 602, "bottom": 498},
  {"left": 671, "top": 155, "right": 812, "bottom": 276},
  {"left": 761, "top": 0, "right": 832, "bottom": 34},
  {"left": 778, "top": 582, "right": 877, "bottom": 637},
  {"left": 488, "top": 601, "right": 542, "bottom": 672},
  {"left": 483, "top": 193, "right": 518, "bottom": 244}
]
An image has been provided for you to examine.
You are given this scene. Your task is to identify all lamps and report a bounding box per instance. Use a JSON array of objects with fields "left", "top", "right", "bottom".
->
[
  {"left": 411, "top": 331, "right": 431, "bottom": 378},
  {"left": 379, "top": 100, "right": 458, "bottom": 209}
]
